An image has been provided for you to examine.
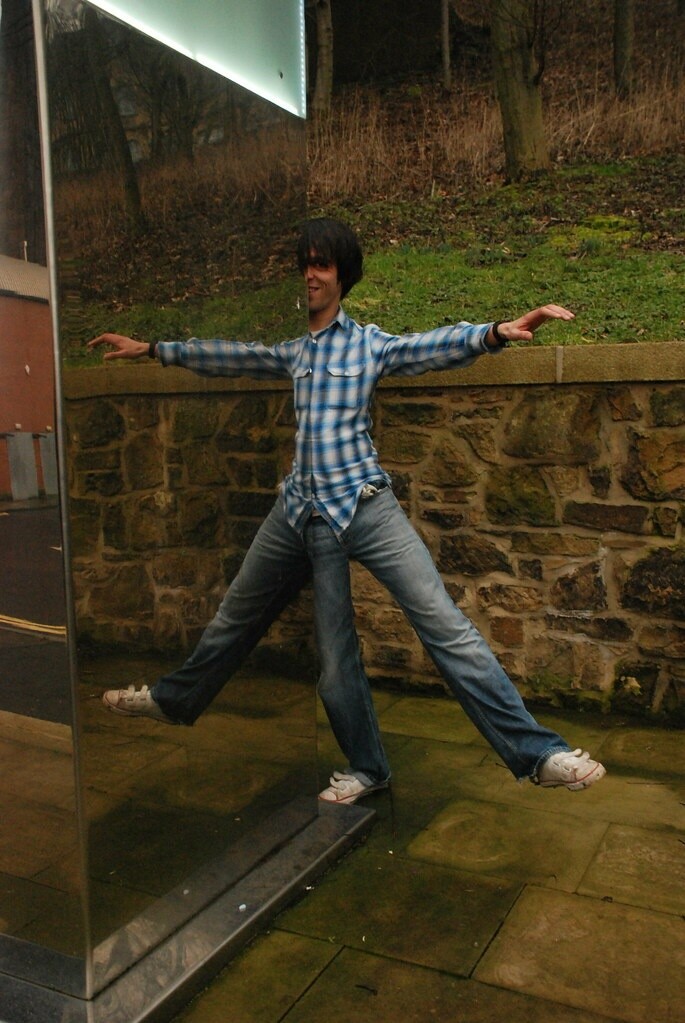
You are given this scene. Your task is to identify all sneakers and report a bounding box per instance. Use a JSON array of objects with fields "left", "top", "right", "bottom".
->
[
  {"left": 104, "top": 683, "right": 182, "bottom": 728},
  {"left": 319, "top": 770, "right": 392, "bottom": 807},
  {"left": 529, "top": 747, "right": 605, "bottom": 793}
]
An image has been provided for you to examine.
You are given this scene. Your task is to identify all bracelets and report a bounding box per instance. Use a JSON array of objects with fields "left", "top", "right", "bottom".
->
[
  {"left": 148, "top": 338, "right": 157, "bottom": 359},
  {"left": 492, "top": 319, "right": 511, "bottom": 342}
]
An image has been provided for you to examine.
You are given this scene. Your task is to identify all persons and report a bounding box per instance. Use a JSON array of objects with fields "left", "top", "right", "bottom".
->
[
  {"left": 86, "top": 334, "right": 307, "bottom": 727},
  {"left": 308, "top": 215, "right": 605, "bottom": 803}
]
